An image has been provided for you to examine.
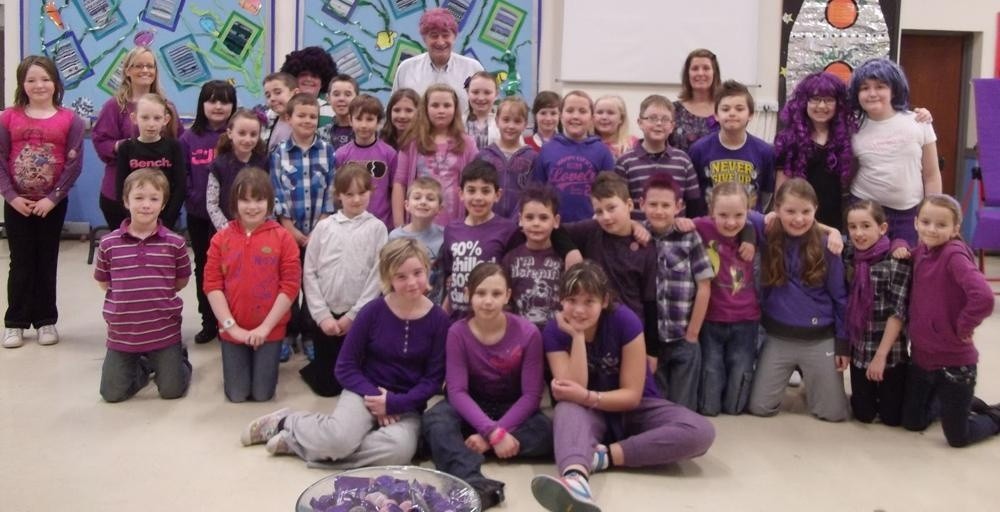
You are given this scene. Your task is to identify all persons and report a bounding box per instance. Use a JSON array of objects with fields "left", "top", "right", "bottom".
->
[
  {"left": 91, "top": 45, "right": 186, "bottom": 231},
  {"left": 685, "top": 78, "right": 776, "bottom": 211},
  {"left": 383, "top": 173, "right": 450, "bottom": 313},
  {"left": 494, "top": 185, "right": 568, "bottom": 332},
  {"left": 260, "top": 69, "right": 301, "bottom": 156},
  {"left": 592, "top": 89, "right": 634, "bottom": 160},
  {"left": 387, "top": 7, "right": 485, "bottom": 121},
  {"left": 333, "top": 91, "right": 400, "bottom": 231},
  {"left": 260, "top": 92, "right": 335, "bottom": 362},
  {"left": 666, "top": 46, "right": 730, "bottom": 149},
  {"left": 479, "top": 95, "right": 539, "bottom": 216},
  {"left": 900, "top": 192, "right": 999, "bottom": 447},
  {"left": 91, "top": 166, "right": 197, "bottom": 402},
  {"left": 180, "top": 75, "right": 252, "bottom": 344},
  {"left": 555, "top": 170, "right": 655, "bottom": 331},
  {"left": 775, "top": 67, "right": 859, "bottom": 225},
  {"left": 393, "top": 84, "right": 482, "bottom": 227},
  {"left": 519, "top": 85, "right": 563, "bottom": 150},
  {"left": 326, "top": 76, "right": 361, "bottom": 148},
  {"left": 382, "top": 82, "right": 422, "bottom": 153},
  {"left": 197, "top": 165, "right": 304, "bottom": 403},
  {"left": 1, "top": 54, "right": 82, "bottom": 348},
  {"left": 846, "top": 57, "right": 944, "bottom": 245},
  {"left": 207, "top": 105, "right": 275, "bottom": 234},
  {"left": 743, "top": 176, "right": 850, "bottom": 422},
  {"left": 427, "top": 260, "right": 559, "bottom": 509},
  {"left": 277, "top": 44, "right": 336, "bottom": 131},
  {"left": 111, "top": 92, "right": 183, "bottom": 231},
  {"left": 298, "top": 164, "right": 387, "bottom": 396},
  {"left": 436, "top": 158, "right": 518, "bottom": 315},
  {"left": 533, "top": 256, "right": 715, "bottom": 512},
  {"left": 635, "top": 173, "right": 714, "bottom": 416},
  {"left": 686, "top": 179, "right": 764, "bottom": 417},
  {"left": 458, "top": 66, "right": 507, "bottom": 154},
  {"left": 826, "top": 192, "right": 910, "bottom": 425},
  {"left": 534, "top": 93, "right": 615, "bottom": 224},
  {"left": 614, "top": 93, "right": 700, "bottom": 214},
  {"left": 232, "top": 229, "right": 455, "bottom": 471}
]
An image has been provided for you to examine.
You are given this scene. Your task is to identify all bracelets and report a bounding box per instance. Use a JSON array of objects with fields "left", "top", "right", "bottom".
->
[
  {"left": 583, "top": 389, "right": 591, "bottom": 408},
  {"left": 591, "top": 390, "right": 599, "bottom": 415}
]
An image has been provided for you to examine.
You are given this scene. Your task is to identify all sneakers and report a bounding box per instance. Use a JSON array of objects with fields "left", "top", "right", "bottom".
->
[
  {"left": 304, "top": 340, "right": 316, "bottom": 361},
  {"left": 194, "top": 323, "right": 218, "bottom": 344},
  {"left": 37, "top": 324, "right": 59, "bottom": 345},
  {"left": 265, "top": 429, "right": 296, "bottom": 456},
  {"left": 531, "top": 474, "right": 601, "bottom": 512},
  {"left": 3, "top": 327, "right": 24, "bottom": 348},
  {"left": 242, "top": 406, "right": 292, "bottom": 446},
  {"left": 584, "top": 445, "right": 608, "bottom": 472},
  {"left": 282, "top": 341, "right": 296, "bottom": 362},
  {"left": 465, "top": 478, "right": 506, "bottom": 511}
]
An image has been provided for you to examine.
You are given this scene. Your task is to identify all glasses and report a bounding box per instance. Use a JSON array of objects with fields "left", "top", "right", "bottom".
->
[
  {"left": 972, "top": 397, "right": 989, "bottom": 411},
  {"left": 129, "top": 63, "right": 156, "bottom": 70},
  {"left": 642, "top": 116, "right": 675, "bottom": 125}
]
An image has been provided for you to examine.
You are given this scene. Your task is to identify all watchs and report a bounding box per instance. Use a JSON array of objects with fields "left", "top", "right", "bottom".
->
[
  {"left": 489, "top": 429, "right": 507, "bottom": 445},
  {"left": 219, "top": 317, "right": 235, "bottom": 335}
]
